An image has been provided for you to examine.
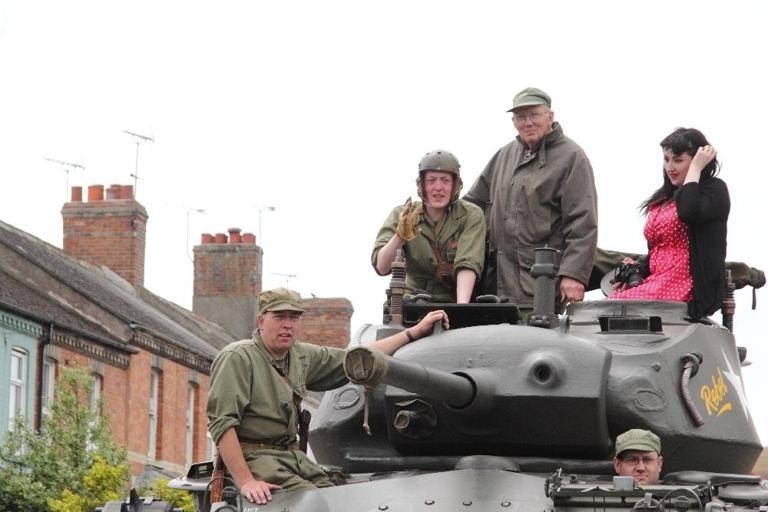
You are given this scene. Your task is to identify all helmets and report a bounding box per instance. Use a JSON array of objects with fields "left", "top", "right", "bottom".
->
[{"left": 415, "top": 149, "right": 464, "bottom": 209}]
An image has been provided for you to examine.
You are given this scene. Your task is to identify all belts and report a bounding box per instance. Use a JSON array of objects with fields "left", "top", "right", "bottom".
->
[{"left": 237, "top": 441, "right": 300, "bottom": 452}]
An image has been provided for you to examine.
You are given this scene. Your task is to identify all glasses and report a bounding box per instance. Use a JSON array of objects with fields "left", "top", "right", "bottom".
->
[{"left": 510, "top": 110, "right": 550, "bottom": 123}]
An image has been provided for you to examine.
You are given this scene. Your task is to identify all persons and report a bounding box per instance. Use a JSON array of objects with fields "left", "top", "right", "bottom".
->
[
  {"left": 207, "top": 290, "right": 450, "bottom": 504},
  {"left": 615, "top": 427, "right": 664, "bottom": 487},
  {"left": 371, "top": 151, "right": 486, "bottom": 305},
  {"left": 463, "top": 88, "right": 597, "bottom": 327},
  {"left": 601, "top": 129, "right": 730, "bottom": 320}
]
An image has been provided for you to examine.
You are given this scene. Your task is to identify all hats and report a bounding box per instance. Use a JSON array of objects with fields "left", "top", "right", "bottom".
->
[
  {"left": 614, "top": 427, "right": 663, "bottom": 460},
  {"left": 256, "top": 286, "right": 306, "bottom": 315},
  {"left": 504, "top": 86, "right": 552, "bottom": 113}
]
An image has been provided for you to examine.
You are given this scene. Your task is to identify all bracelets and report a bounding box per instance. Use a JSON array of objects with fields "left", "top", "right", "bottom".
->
[{"left": 404, "top": 329, "right": 414, "bottom": 342}]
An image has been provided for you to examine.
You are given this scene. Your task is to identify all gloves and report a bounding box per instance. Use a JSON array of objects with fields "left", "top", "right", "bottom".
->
[{"left": 395, "top": 195, "right": 427, "bottom": 241}]
